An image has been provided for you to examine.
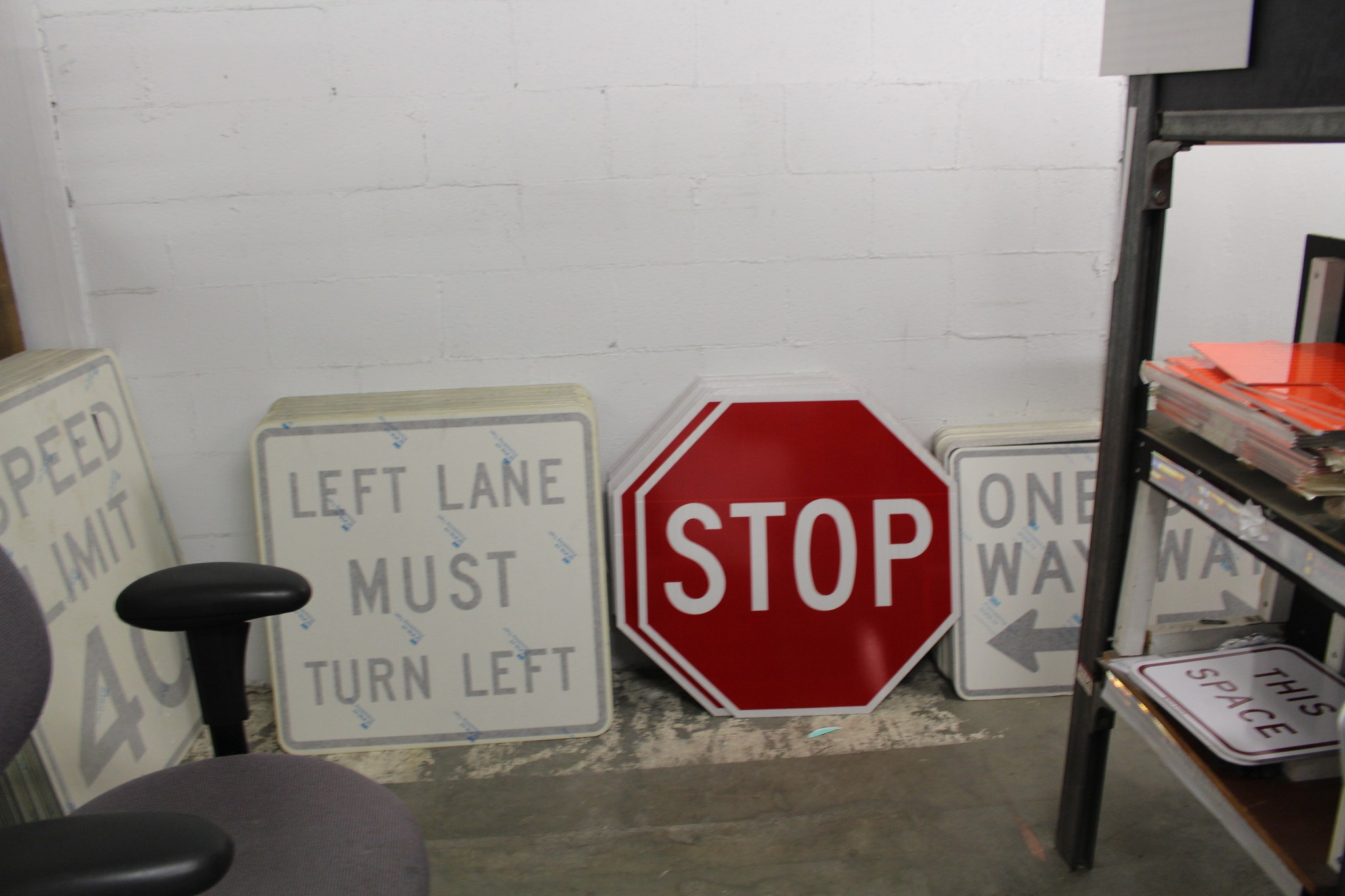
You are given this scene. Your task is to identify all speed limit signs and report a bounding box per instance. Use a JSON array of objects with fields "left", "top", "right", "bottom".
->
[{"left": 2, "top": 349, "right": 207, "bottom": 813}]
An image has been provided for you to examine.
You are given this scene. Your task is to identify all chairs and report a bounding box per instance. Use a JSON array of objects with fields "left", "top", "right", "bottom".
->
[{"left": 0, "top": 539, "right": 431, "bottom": 895}]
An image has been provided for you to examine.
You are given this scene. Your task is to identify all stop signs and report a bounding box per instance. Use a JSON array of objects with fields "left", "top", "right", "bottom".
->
[{"left": 633, "top": 400, "right": 964, "bottom": 717}]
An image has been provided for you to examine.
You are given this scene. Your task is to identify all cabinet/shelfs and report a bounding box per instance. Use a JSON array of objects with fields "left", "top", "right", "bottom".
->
[{"left": 1055, "top": 0, "right": 1345, "bottom": 896}]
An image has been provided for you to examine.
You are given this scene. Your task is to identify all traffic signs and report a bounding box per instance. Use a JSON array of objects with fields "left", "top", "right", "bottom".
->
[
  {"left": 948, "top": 440, "right": 1277, "bottom": 698},
  {"left": 252, "top": 408, "right": 614, "bottom": 757},
  {"left": 1129, "top": 638, "right": 1345, "bottom": 759}
]
